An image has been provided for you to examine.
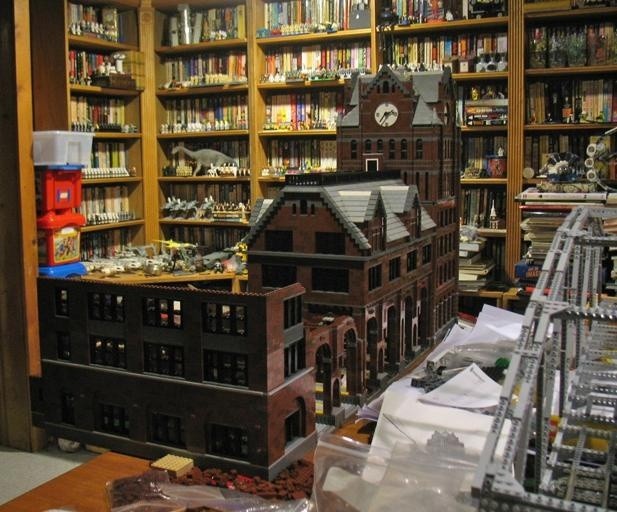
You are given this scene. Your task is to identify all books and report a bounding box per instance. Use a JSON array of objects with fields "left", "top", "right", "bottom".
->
[
  {"left": 386, "top": 0, "right": 508, "bottom": 75},
  {"left": 456, "top": 83, "right": 507, "bottom": 282},
  {"left": 515, "top": 20, "right": 617, "bottom": 292},
  {"left": 164, "top": 4, "right": 251, "bottom": 255},
  {"left": 68, "top": 0, "right": 132, "bottom": 263},
  {"left": 263, "top": 0, "right": 371, "bottom": 172}
]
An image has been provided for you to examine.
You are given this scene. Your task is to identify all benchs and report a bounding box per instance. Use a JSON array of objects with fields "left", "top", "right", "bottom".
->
[
  {"left": 503, "top": 287, "right": 535, "bottom": 313},
  {"left": 458, "top": 284, "right": 506, "bottom": 307}
]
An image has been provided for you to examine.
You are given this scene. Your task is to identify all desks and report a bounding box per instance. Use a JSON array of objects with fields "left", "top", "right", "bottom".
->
[{"left": 84, "top": 258, "right": 254, "bottom": 283}]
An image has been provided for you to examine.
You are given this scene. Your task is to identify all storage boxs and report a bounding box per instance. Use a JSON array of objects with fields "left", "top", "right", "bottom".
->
[{"left": 31, "top": 130, "right": 95, "bottom": 276}]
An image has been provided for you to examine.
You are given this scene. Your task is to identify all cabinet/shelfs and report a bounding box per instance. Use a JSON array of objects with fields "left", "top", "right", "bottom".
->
[{"left": 31, "top": 0, "right": 617, "bottom": 302}]
